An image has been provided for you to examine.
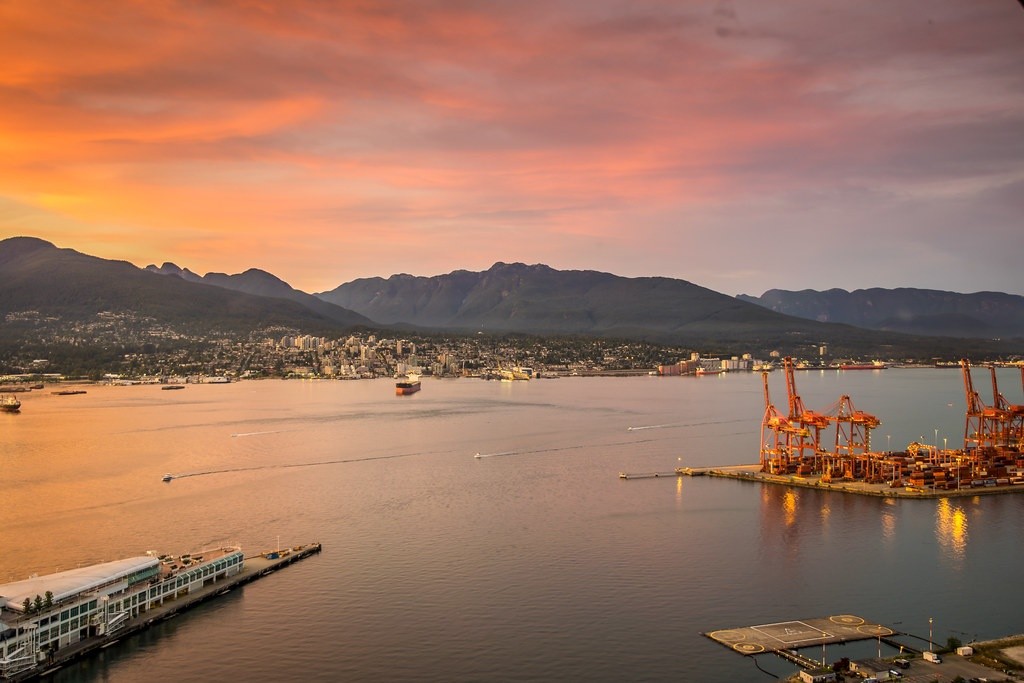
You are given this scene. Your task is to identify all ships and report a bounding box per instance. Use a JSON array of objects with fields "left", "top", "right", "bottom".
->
[
  {"left": 0, "top": 541, "right": 322, "bottom": 683},
  {"left": 396, "top": 374, "right": 422, "bottom": 396}
]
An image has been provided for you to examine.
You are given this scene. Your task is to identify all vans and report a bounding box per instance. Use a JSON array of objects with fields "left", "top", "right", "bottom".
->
[{"left": 894, "top": 659, "right": 910, "bottom": 669}]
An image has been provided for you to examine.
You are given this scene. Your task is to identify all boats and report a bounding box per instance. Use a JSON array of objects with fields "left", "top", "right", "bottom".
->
[
  {"left": 627, "top": 426, "right": 632, "bottom": 431},
  {"left": 1, "top": 395, "right": 21, "bottom": 412},
  {"left": 162, "top": 473, "right": 172, "bottom": 481},
  {"left": 231, "top": 433, "right": 238, "bottom": 438},
  {"left": 50, "top": 390, "right": 88, "bottom": 396},
  {"left": 162, "top": 386, "right": 184, "bottom": 390},
  {"left": 474, "top": 453, "right": 481, "bottom": 458}
]
